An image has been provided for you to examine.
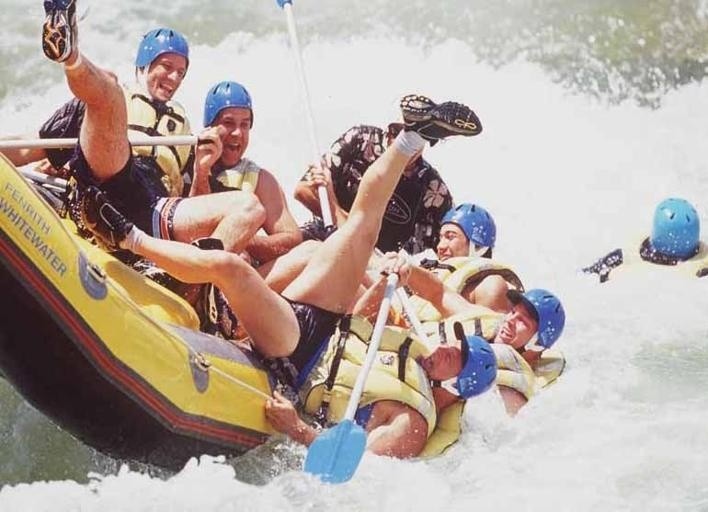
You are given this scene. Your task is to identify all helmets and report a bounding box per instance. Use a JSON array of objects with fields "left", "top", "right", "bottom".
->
[
  {"left": 646, "top": 197, "right": 702, "bottom": 259},
  {"left": 450, "top": 320, "right": 499, "bottom": 400},
  {"left": 436, "top": 201, "right": 499, "bottom": 251},
  {"left": 134, "top": 25, "right": 191, "bottom": 71},
  {"left": 203, "top": 80, "right": 254, "bottom": 129},
  {"left": 503, "top": 287, "right": 567, "bottom": 349}
]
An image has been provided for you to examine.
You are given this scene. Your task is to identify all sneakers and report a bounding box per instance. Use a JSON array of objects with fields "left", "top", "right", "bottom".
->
[
  {"left": 39, "top": 1, "right": 81, "bottom": 63},
  {"left": 79, "top": 182, "right": 135, "bottom": 256},
  {"left": 400, "top": 93, "right": 484, "bottom": 147}
]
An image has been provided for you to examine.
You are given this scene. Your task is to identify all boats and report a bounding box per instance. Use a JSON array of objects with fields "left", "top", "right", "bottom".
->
[{"left": 0, "top": 136, "right": 557, "bottom": 471}]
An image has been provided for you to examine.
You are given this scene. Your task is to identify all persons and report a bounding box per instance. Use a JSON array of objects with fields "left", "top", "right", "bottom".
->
[
  {"left": 186, "top": 80, "right": 304, "bottom": 264},
  {"left": 2, "top": 1, "right": 269, "bottom": 251},
  {"left": 80, "top": 94, "right": 564, "bottom": 460},
  {"left": 582, "top": 198, "right": 708, "bottom": 282}
]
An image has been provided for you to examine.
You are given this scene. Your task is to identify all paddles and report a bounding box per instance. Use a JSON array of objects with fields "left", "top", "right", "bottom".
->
[{"left": 302, "top": 271, "right": 401, "bottom": 485}]
